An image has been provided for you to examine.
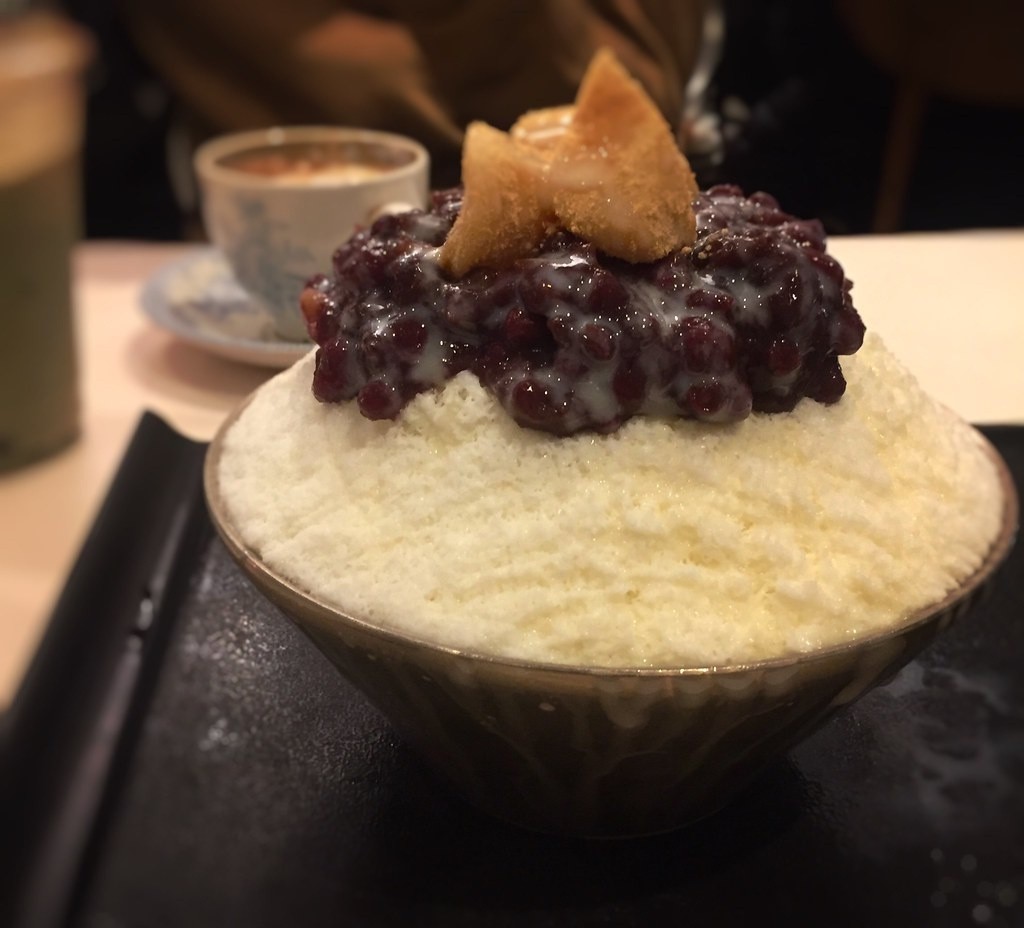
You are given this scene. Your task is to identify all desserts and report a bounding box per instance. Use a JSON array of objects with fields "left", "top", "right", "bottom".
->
[{"left": 201, "top": 43, "right": 1010, "bottom": 673}]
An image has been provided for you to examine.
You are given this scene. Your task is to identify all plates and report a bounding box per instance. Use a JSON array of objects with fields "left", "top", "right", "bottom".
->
[{"left": 141, "top": 244, "right": 317, "bottom": 369}]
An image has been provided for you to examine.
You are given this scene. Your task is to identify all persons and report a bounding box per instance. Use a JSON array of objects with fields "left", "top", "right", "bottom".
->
[{"left": 188, "top": 1, "right": 710, "bottom": 214}]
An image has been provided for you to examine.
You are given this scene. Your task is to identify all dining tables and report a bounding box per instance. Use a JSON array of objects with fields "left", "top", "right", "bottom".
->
[{"left": 0, "top": 228, "right": 1024, "bottom": 928}]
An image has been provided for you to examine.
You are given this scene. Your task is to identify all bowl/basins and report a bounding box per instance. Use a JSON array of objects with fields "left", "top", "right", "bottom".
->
[{"left": 197, "top": 374, "right": 1022, "bottom": 802}]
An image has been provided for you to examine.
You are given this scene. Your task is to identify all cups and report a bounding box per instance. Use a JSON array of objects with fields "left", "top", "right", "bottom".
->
[
  {"left": 194, "top": 125, "right": 432, "bottom": 341},
  {"left": 1, "top": 9, "right": 93, "bottom": 473}
]
